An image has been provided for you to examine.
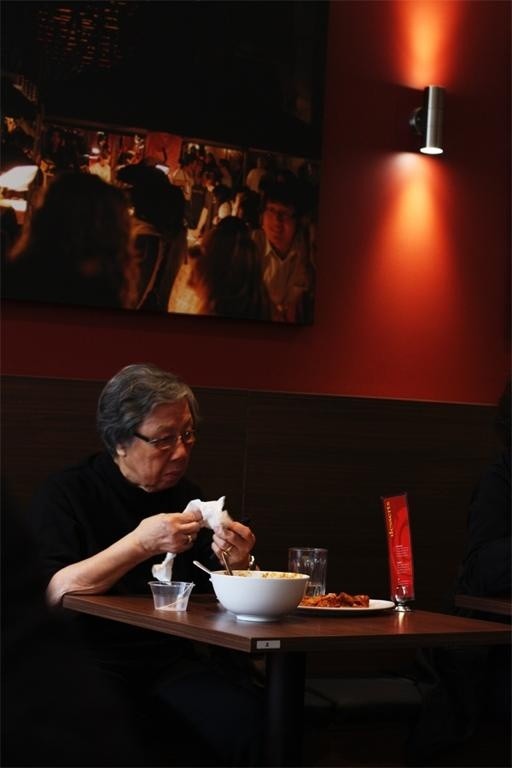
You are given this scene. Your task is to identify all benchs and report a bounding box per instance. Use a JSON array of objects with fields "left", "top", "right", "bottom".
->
[{"left": 0, "top": 377, "right": 512, "bottom": 767}]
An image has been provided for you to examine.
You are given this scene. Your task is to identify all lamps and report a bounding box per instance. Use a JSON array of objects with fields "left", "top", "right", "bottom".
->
[{"left": 404, "top": 81, "right": 454, "bottom": 154}]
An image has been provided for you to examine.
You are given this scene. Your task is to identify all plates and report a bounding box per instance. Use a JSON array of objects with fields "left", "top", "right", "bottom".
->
[{"left": 295, "top": 597, "right": 399, "bottom": 611}]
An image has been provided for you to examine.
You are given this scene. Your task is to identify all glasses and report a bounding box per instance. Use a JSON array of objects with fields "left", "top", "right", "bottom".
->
[{"left": 126, "top": 428, "right": 198, "bottom": 451}]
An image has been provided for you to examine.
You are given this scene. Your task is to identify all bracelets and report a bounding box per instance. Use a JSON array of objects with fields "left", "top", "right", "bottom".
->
[{"left": 247, "top": 552, "right": 254, "bottom": 570}]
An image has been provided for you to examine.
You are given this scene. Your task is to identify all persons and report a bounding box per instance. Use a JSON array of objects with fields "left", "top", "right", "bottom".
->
[
  {"left": 0, "top": 117, "right": 316, "bottom": 320},
  {"left": 33, "top": 364, "right": 262, "bottom": 615}
]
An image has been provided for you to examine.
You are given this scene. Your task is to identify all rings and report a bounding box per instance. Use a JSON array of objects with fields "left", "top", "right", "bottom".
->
[
  {"left": 186, "top": 534, "right": 192, "bottom": 543},
  {"left": 225, "top": 545, "right": 235, "bottom": 552}
]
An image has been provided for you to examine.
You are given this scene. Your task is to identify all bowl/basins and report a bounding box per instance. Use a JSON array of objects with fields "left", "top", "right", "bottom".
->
[{"left": 209, "top": 570, "right": 311, "bottom": 622}]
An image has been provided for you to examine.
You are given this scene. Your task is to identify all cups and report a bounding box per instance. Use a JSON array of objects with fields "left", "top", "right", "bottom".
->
[
  {"left": 148, "top": 580, "right": 196, "bottom": 612},
  {"left": 288, "top": 546, "right": 330, "bottom": 597}
]
